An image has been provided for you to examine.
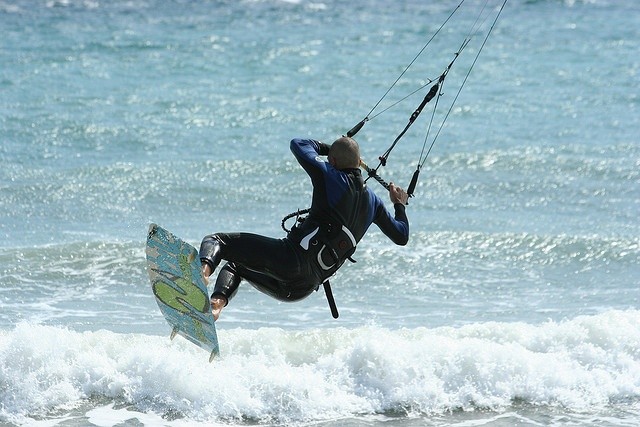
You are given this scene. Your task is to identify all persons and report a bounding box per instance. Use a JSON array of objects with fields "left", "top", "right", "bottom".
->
[{"left": 197, "top": 136, "right": 411, "bottom": 322}]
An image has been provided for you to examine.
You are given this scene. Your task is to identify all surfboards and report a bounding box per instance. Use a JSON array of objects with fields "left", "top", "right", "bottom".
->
[{"left": 146, "top": 223, "right": 218, "bottom": 363}]
